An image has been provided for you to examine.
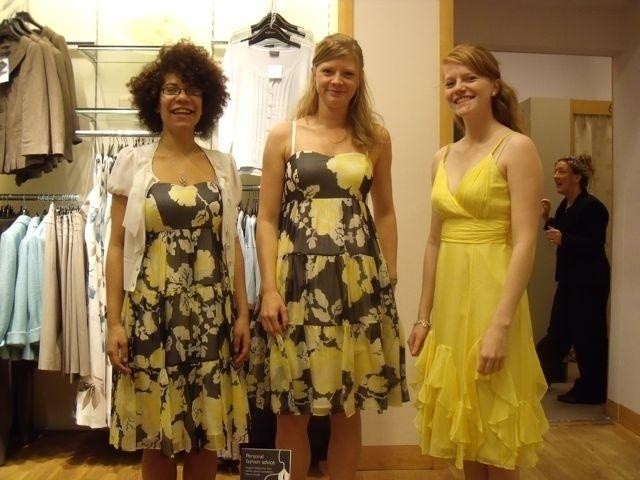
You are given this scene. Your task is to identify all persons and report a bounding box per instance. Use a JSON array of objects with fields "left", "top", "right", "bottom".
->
[
  {"left": 536, "top": 153, "right": 610, "bottom": 405},
  {"left": 407, "top": 41, "right": 552, "bottom": 480},
  {"left": 253, "top": 30, "right": 411, "bottom": 480},
  {"left": 102, "top": 37, "right": 252, "bottom": 480}
]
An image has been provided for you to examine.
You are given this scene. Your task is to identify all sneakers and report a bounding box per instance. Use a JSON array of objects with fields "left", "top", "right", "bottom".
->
[{"left": 558, "top": 385, "right": 585, "bottom": 402}]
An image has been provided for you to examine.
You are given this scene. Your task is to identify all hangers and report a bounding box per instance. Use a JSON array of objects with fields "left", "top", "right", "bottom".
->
[
  {"left": 242, "top": 2, "right": 304, "bottom": 48},
  {"left": 236, "top": 190, "right": 259, "bottom": 237},
  {"left": 86, "top": 135, "right": 163, "bottom": 164},
  {"left": 0, "top": 193, "right": 80, "bottom": 233},
  {"left": 0, "top": 11, "right": 43, "bottom": 41}
]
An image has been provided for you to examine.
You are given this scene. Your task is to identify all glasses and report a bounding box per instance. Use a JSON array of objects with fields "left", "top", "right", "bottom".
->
[{"left": 160, "top": 86, "right": 205, "bottom": 97}]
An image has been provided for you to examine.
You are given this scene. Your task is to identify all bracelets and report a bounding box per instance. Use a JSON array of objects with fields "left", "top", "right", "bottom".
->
[{"left": 411, "top": 319, "right": 434, "bottom": 330}]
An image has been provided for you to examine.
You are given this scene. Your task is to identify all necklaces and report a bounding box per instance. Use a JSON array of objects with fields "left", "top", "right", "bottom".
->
[{"left": 159, "top": 140, "right": 201, "bottom": 186}]
